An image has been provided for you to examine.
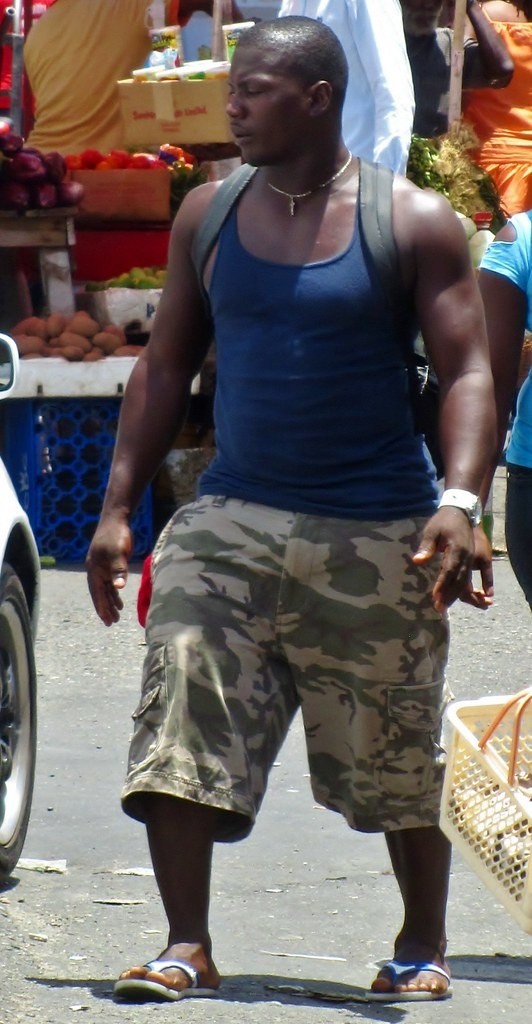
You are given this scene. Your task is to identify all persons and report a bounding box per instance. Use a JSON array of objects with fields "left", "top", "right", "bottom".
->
[
  {"left": 22, "top": 0, "right": 157, "bottom": 160},
  {"left": 85, "top": 15, "right": 499, "bottom": 1003},
  {"left": 462, "top": 0, "right": 532, "bottom": 219},
  {"left": 278, "top": 0, "right": 416, "bottom": 179},
  {"left": 175, "top": 0, "right": 213, "bottom": 61},
  {"left": 397, "top": 0, "right": 515, "bottom": 137},
  {"left": 476, "top": 208, "right": 532, "bottom": 611}
]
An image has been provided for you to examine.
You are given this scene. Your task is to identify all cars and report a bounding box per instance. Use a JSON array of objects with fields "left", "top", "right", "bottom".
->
[{"left": 0, "top": 331, "right": 44, "bottom": 878}]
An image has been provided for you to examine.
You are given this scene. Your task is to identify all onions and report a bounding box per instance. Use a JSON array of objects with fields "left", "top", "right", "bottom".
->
[{"left": 0, "top": 134, "right": 84, "bottom": 209}]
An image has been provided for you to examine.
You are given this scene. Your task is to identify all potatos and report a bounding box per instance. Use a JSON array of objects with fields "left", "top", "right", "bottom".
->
[{"left": 10, "top": 310, "right": 146, "bottom": 362}]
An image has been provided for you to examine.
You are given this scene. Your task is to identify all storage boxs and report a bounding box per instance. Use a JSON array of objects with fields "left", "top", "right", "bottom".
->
[
  {"left": 117, "top": 77, "right": 232, "bottom": 143},
  {"left": 65, "top": 168, "right": 181, "bottom": 282},
  {"left": 0, "top": 356, "right": 201, "bottom": 400},
  {"left": 74, "top": 288, "right": 216, "bottom": 353}
]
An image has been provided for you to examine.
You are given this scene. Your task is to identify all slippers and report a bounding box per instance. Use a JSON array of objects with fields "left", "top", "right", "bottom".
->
[
  {"left": 365, "top": 959, "right": 455, "bottom": 1001},
  {"left": 114, "top": 958, "right": 222, "bottom": 1001}
]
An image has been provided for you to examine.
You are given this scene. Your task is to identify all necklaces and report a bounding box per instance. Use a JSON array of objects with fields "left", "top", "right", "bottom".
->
[{"left": 267, "top": 149, "right": 353, "bottom": 216}]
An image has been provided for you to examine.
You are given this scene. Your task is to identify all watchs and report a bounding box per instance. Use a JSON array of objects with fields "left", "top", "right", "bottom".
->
[{"left": 437, "top": 488, "right": 484, "bottom": 528}]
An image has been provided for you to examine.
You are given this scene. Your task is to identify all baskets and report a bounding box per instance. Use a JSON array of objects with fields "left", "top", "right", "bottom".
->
[{"left": 438, "top": 687, "right": 532, "bottom": 934}]
